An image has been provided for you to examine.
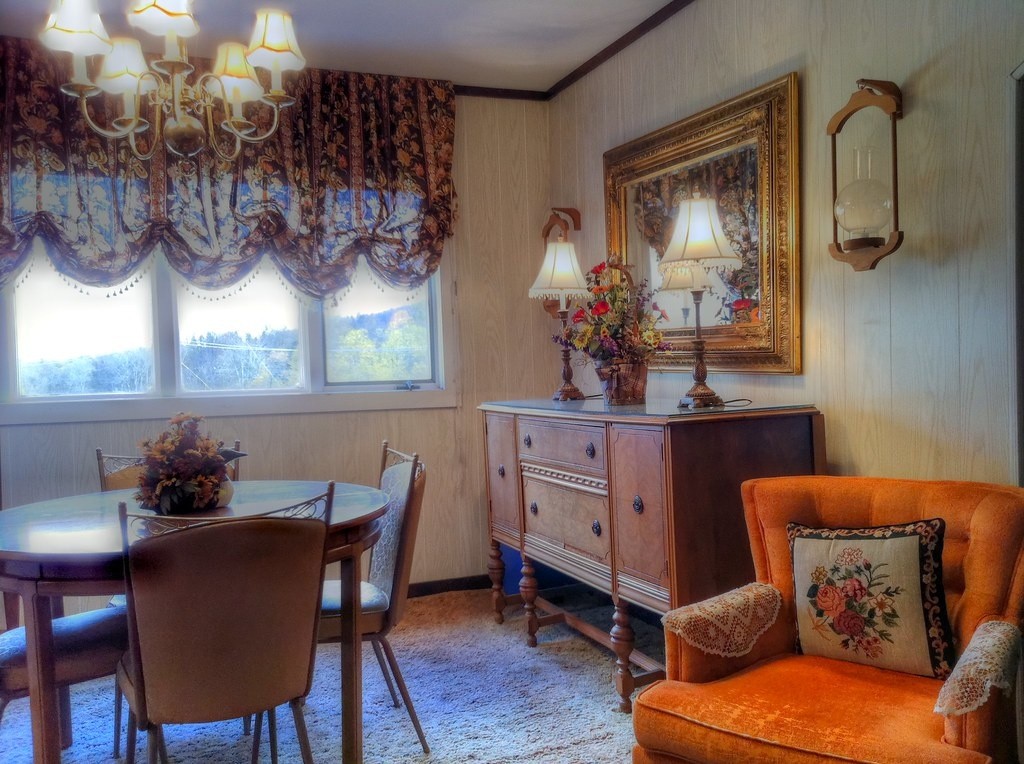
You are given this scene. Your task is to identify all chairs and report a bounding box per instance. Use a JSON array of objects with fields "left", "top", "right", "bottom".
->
[
  {"left": 245, "top": 438, "right": 433, "bottom": 764},
  {"left": 113, "top": 480, "right": 334, "bottom": 764},
  {"left": 96, "top": 439, "right": 246, "bottom": 487},
  {"left": 0, "top": 591, "right": 171, "bottom": 764}
]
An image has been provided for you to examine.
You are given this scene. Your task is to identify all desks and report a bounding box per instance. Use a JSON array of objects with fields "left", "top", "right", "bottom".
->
[
  {"left": 475, "top": 398, "right": 825, "bottom": 717},
  {"left": 0, "top": 479, "right": 393, "bottom": 764}
]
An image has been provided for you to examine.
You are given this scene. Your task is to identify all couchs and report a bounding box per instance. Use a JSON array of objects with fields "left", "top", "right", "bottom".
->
[{"left": 633, "top": 472, "right": 1024, "bottom": 764}]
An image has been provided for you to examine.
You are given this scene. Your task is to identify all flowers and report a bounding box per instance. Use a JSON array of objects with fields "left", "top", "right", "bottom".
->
[
  {"left": 553, "top": 267, "right": 674, "bottom": 374},
  {"left": 129, "top": 416, "right": 249, "bottom": 516}
]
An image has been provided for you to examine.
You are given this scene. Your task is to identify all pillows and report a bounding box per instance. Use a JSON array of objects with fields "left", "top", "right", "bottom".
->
[{"left": 786, "top": 519, "right": 957, "bottom": 680}]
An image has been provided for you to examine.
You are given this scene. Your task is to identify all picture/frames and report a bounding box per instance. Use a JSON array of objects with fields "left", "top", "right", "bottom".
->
[{"left": 601, "top": 70, "right": 802, "bottom": 377}]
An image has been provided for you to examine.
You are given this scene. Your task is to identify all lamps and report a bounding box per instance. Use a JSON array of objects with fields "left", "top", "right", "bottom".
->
[
  {"left": 528, "top": 235, "right": 594, "bottom": 403},
  {"left": 658, "top": 263, "right": 716, "bottom": 325},
  {"left": 36, "top": 1, "right": 307, "bottom": 163},
  {"left": 654, "top": 190, "right": 743, "bottom": 409}
]
